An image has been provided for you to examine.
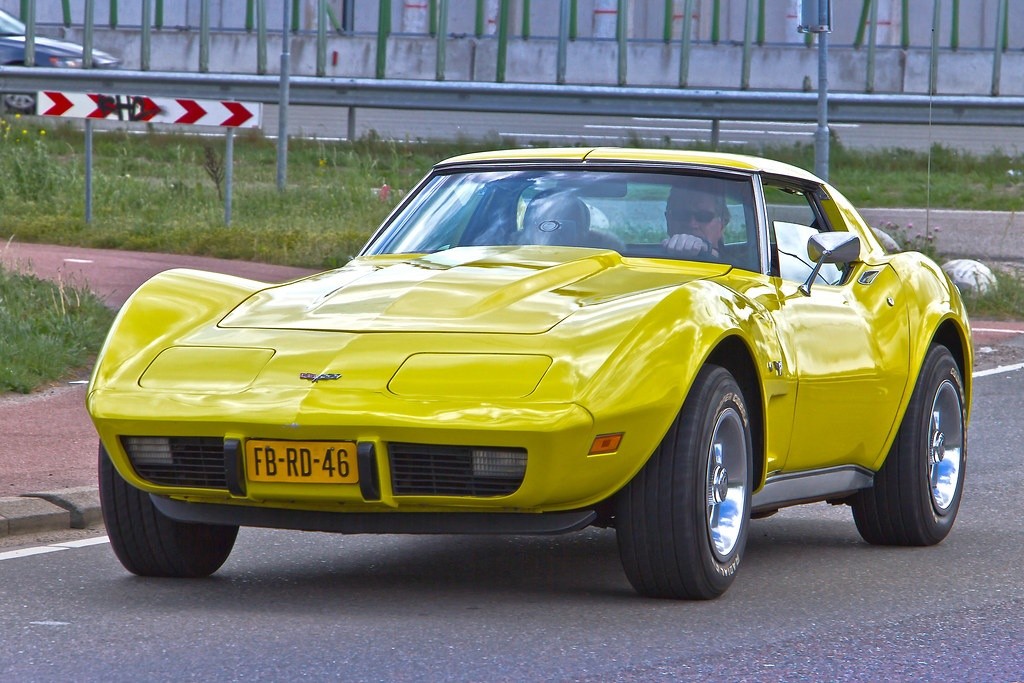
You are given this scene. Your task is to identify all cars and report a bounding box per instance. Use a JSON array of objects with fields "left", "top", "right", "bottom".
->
[{"left": 0, "top": 10, "right": 118, "bottom": 114}]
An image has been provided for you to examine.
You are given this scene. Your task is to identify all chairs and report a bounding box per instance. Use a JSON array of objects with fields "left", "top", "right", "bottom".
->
[{"left": 508, "top": 189, "right": 626, "bottom": 255}]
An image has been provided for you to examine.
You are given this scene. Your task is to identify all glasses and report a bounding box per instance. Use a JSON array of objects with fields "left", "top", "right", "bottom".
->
[{"left": 665, "top": 209, "right": 718, "bottom": 223}]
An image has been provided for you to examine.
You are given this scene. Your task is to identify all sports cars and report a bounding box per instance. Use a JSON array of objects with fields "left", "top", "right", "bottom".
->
[{"left": 86, "top": 147, "right": 974, "bottom": 599}]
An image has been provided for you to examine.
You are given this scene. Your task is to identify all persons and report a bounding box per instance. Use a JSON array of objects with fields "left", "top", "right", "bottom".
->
[{"left": 659, "top": 174, "right": 730, "bottom": 262}]
